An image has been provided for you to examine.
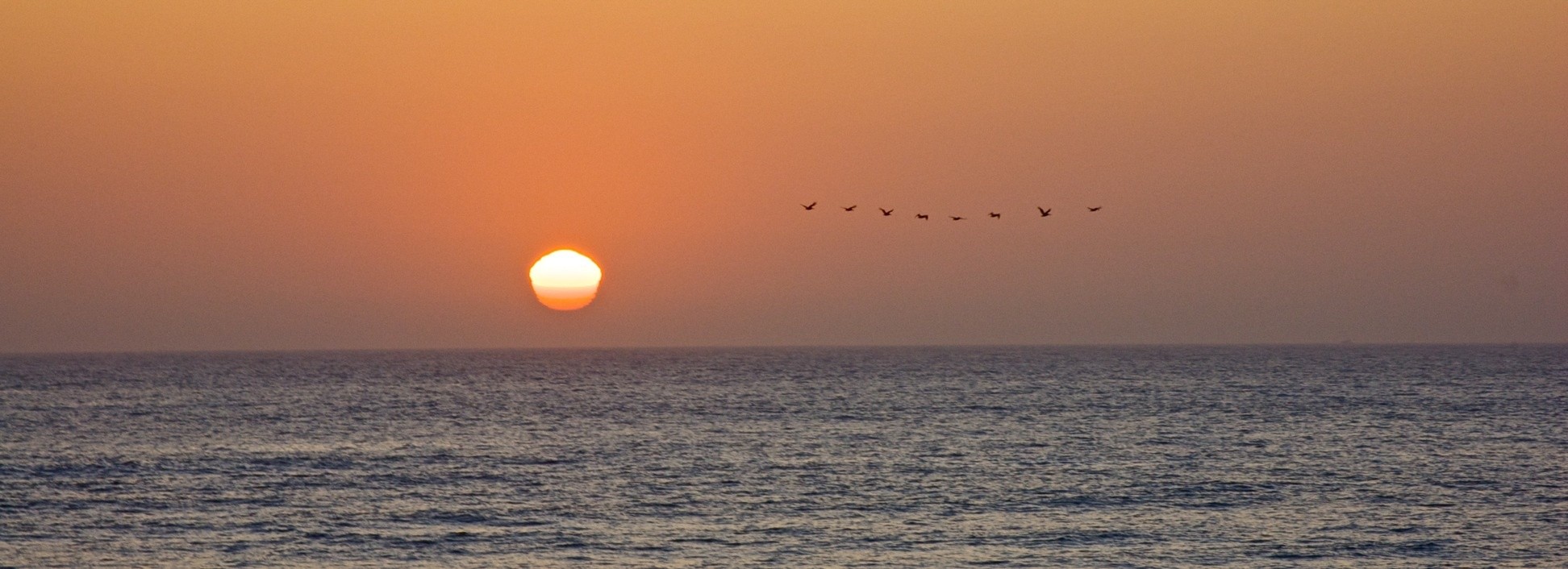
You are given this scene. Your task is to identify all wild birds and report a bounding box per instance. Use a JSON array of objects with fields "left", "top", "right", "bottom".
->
[
  {"left": 1037, "top": 206, "right": 1051, "bottom": 217},
  {"left": 840, "top": 205, "right": 857, "bottom": 211},
  {"left": 879, "top": 207, "right": 894, "bottom": 216},
  {"left": 951, "top": 217, "right": 967, "bottom": 220},
  {"left": 988, "top": 213, "right": 1000, "bottom": 219},
  {"left": 800, "top": 201, "right": 817, "bottom": 210},
  {"left": 1087, "top": 206, "right": 1102, "bottom": 212},
  {"left": 915, "top": 213, "right": 928, "bottom": 220}
]
